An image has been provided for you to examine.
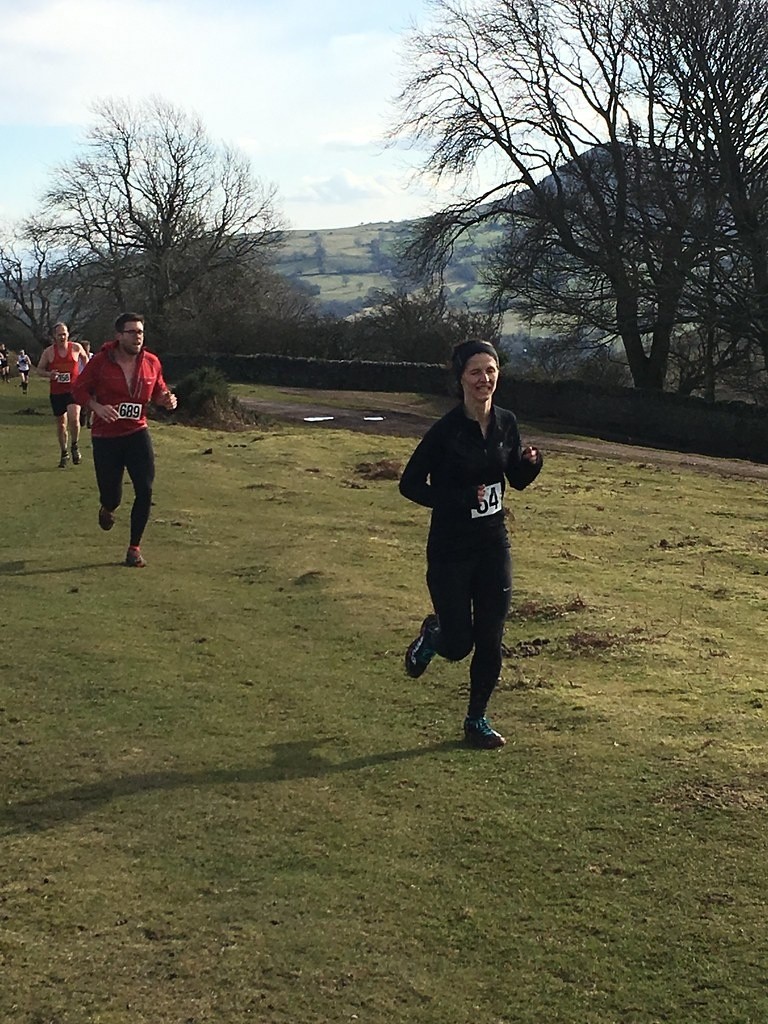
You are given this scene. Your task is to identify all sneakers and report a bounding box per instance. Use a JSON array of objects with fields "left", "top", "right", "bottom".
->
[
  {"left": 99, "top": 505, "right": 115, "bottom": 530},
  {"left": 126, "top": 546, "right": 146, "bottom": 567},
  {"left": 464, "top": 717, "right": 506, "bottom": 747},
  {"left": 405, "top": 614, "right": 436, "bottom": 679}
]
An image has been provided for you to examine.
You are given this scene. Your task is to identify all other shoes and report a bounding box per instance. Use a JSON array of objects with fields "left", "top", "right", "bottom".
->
[
  {"left": 71, "top": 447, "right": 81, "bottom": 465},
  {"left": 87, "top": 423, "right": 92, "bottom": 428},
  {"left": 58, "top": 452, "right": 70, "bottom": 468},
  {"left": 23, "top": 390, "right": 27, "bottom": 394},
  {"left": 80, "top": 416, "right": 86, "bottom": 426},
  {"left": 6, "top": 379, "right": 10, "bottom": 383}
]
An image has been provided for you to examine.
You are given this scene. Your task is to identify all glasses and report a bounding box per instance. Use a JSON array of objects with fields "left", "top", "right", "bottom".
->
[{"left": 121, "top": 329, "right": 144, "bottom": 335}]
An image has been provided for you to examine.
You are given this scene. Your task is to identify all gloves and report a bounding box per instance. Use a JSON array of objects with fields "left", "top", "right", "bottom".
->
[
  {"left": 22, "top": 360, "right": 26, "bottom": 364},
  {"left": 17, "top": 364, "right": 20, "bottom": 367}
]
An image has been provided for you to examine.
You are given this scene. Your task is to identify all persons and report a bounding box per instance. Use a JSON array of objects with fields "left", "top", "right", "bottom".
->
[
  {"left": 70, "top": 313, "right": 177, "bottom": 566},
  {"left": 398, "top": 338, "right": 544, "bottom": 747},
  {"left": 0, "top": 322, "right": 95, "bottom": 467}
]
